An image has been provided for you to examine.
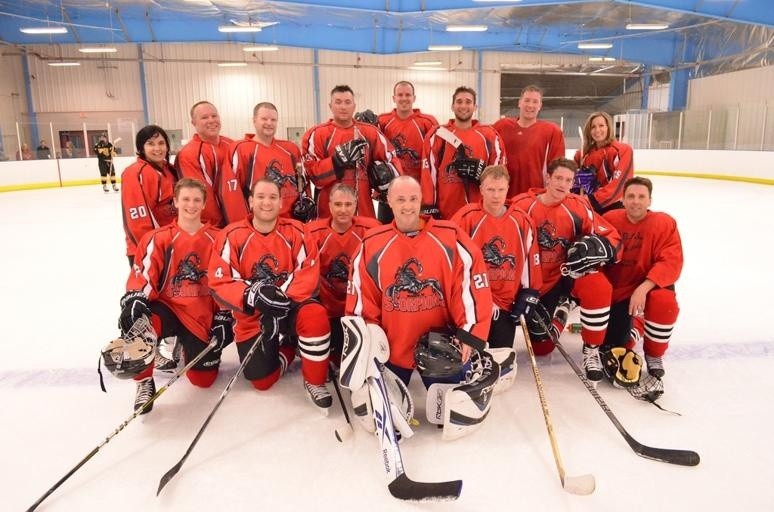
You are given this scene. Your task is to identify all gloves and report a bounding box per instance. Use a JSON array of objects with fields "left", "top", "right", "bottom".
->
[
  {"left": 206, "top": 307, "right": 237, "bottom": 354},
  {"left": 573, "top": 167, "right": 599, "bottom": 196},
  {"left": 240, "top": 280, "right": 295, "bottom": 349},
  {"left": 452, "top": 158, "right": 487, "bottom": 182},
  {"left": 334, "top": 137, "right": 368, "bottom": 170},
  {"left": 422, "top": 204, "right": 441, "bottom": 220},
  {"left": 503, "top": 287, "right": 541, "bottom": 327},
  {"left": 117, "top": 288, "right": 152, "bottom": 330},
  {"left": 293, "top": 196, "right": 316, "bottom": 222},
  {"left": 368, "top": 160, "right": 401, "bottom": 206},
  {"left": 560, "top": 233, "right": 616, "bottom": 282}
]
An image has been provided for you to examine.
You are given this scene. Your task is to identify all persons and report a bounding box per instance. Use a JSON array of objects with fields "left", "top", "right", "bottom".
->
[
  {"left": 16, "top": 143, "right": 36, "bottom": 161},
  {"left": 61, "top": 134, "right": 75, "bottom": 158},
  {"left": 342, "top": 176, "right": 493, "bottom": 439},
  {"left": 121, "top": 81, "right": 683, "bottom": 416},
  {"left": 93, "top": 133, "right": 121, "bottom": 192},
  {"left": 37, "top": 139, "right": 53, "bottom": 159}
]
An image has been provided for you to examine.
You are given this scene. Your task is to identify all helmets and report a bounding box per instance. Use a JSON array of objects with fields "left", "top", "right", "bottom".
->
[
  {"left": 412, "top": 328, "right": 474, "bottom": 392},
  {"left": 100, "top": 314, "right": 161, "bottom": 381}
]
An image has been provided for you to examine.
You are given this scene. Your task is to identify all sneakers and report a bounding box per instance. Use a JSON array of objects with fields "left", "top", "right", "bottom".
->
[
  {"left": 102, "top": 184, "right": 119, "bottom": 192},
  {"left": 580, "top": 343, "right": 667, "bottom": 382},
  {"left": 132, "top": 350, "right": 178, "bottom": 415},
  {"left": 302, "top": 379, "right": 335, "bottom": 410},
  {"left": 552, "top": 294, "right": 577, "bottom": 330}
]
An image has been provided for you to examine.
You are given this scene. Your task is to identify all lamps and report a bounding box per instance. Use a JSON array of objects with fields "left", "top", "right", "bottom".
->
[
  {"left": 79, "top": 1, "right": 117, "bottom": 54},
  {"left": 577, "top": 17, "right": 613, "bottom": 50},
  {"left": 218, "top": 13, "right": 262, "bottom": 33},
  {"left": 428, "top": 24, "right": 463, "bottom": 50},
  {"left": 588, "top": 39, "right": 616, "bottom": 62},
  {"left": 243, "top": 15, "right": 277, "bottom": 52},
  {"left": 19, "top": 0, "right": 67, "bottom": 34},
  {"left": 626, "top": 2, "right": 669, "bottom": 31},
  {"left": 47, "top": 42, "right": 81, "bottom": 66},
  {"left": 446, "top": 23, "right": 488, "bottom": 32}
]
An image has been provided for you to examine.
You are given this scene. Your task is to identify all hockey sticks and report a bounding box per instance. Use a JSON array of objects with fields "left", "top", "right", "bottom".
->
[
  {"left": 435, "top": 126, "right": 469, "bottom": 205},
  {"left": 156, "top": 336, "right": 260, "bottom": 497},
  {"left": 577, "top": 126, "right": 585, "bottom": 194},
  {"left": 521, "top": 314, "right": 596, "bottom": 496},
  {"left": 535, "top": 310, "right": 700, "bottom": 466},
  {"left": 109, "top": 137, "right": 121, "bottom": 186},
  {"left": 369, "top": 376, "right": 462, "bottom": 500},
  {"left": 328, "top": 361, "right": 355, "bottom": 443}
]
